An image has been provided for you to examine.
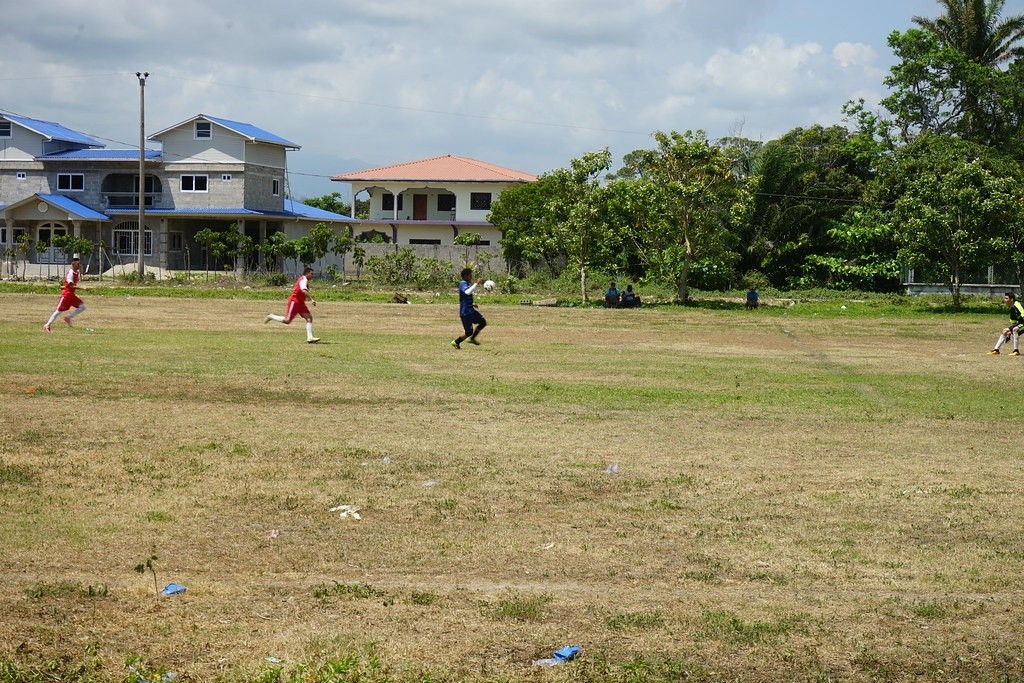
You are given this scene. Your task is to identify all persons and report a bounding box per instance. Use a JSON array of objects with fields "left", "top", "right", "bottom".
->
[
  {"left": 986, "top": 292, "right": 1024, "bottom": 355},
  {"left": 451, "top": 268, "right": 487, "bottom": 349},
  {"left": 264, "top": 268, "right": 321, "bottom": 343},
  {"left": 745, "top": 287, "right": 758, "bottom": 311},
  {"left": 620, "top": 285, "right": 640, "bottom": 310},
  {"left": 43, "top": 258, "right": 86, "bottom": 334},
  {"left": 605, "top": 282, "right": 620, "bottom": 308}
]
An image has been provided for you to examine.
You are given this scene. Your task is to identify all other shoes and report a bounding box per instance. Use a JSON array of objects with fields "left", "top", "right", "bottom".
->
[
  {"left": 467, "top": 337, "right": 479, "bottom": 345},
  {"left": 263, "top": 316, "right": 271, "bottom": 324},
  {"left": 451, "top": 340, "right": 460, "bottom": 349},
  {"left": 44, "top": 325, "right": 50, "bottom": 333},
  {"left": 987, "top": 349, "right": 1000, "bottom": 355},
  {"left": 64, "top": 317, "right": 72, "bottom": 326},
  {"left": 1008, "top": 352, "right": 1020, "bottom": 355},
  {"left": 307, "top": 337, "right": 321, "bottom": 343}
]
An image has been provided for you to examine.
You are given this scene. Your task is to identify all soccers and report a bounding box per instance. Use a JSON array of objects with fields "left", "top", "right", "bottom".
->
[{"left": 484, "top": 280, "right": 496, "bottom": 293}]
